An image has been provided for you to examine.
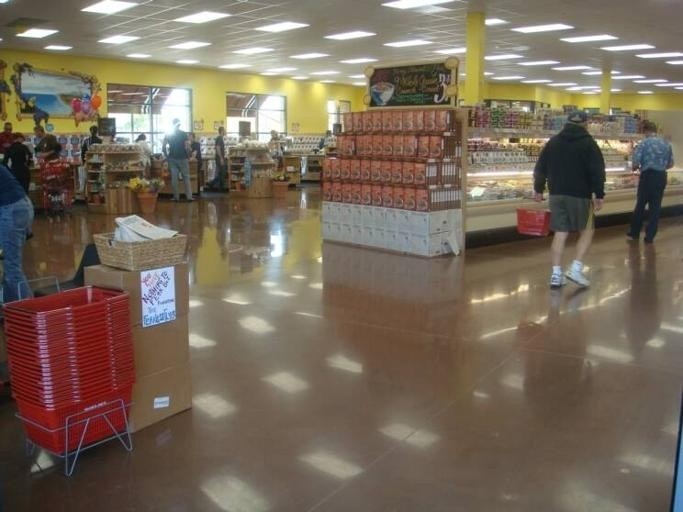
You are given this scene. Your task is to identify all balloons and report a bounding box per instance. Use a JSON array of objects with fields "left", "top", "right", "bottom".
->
[{"left": 72, "top": 93, "right": 101, "bottom": 115}]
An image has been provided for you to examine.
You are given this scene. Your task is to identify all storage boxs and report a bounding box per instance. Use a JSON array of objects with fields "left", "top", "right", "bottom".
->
[
  {"left": 82, "top": 265, "right": 189, "bottom": 329},
  {"left": 127, "top": 365, "right": 192, "bottom": 435},
  {"left": 129, "top": 316, "right": 189, "bottom": 377}
]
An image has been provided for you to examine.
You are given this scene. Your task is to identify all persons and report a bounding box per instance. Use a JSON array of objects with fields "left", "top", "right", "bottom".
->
[
  {"left": 0, "top": 164, "right": 34, "bottom": 301},
  {"left": 533, "top": 110, "right": 606, "bottom": 288},
  {"left": 269, "top": 130, "right": 279, "bottom": 140},
  {"left": 0, "top": 122, "right": 226, "bottom": 201},
  {"left": 319, "top": 130, "right": 332, "bottom": 148},
  {"left": 625, "top": 121, "right": 674, "bottom": 244}
]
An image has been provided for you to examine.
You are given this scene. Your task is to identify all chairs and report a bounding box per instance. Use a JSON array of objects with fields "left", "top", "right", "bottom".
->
[{"left": 33, "top": 243, "right": 100, "bottom": 297}]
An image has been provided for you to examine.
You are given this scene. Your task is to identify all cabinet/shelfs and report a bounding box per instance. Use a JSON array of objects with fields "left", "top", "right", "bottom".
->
[
  {"left": 84, "top": 151, "right": 144, "bottom": 213},
  {"left": 466, "top": 109, "right": 683, "bottom": 248},
  {"left": 320, "top": 107, "right": 461, "bottom": 258},
  {"left": 227, "top": 146, "right": 275, "bottom": 198}
]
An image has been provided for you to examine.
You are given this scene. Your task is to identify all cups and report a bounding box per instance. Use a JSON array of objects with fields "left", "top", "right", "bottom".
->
[{"left": 371, "top": 82, "right": 397, "bottom": 105}]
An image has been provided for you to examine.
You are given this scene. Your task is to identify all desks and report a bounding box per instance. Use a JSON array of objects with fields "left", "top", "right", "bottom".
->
[{"left": 28, "top": 162, "right": 80, "bottom": 209}]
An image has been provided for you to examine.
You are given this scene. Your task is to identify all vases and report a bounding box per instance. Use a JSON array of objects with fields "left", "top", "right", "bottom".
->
[
  {"left": 137, "top": 192, "right": 158, "bottom": 213},
  {"left": 273, "top": 181, "right": 289, "bottom": 196}
]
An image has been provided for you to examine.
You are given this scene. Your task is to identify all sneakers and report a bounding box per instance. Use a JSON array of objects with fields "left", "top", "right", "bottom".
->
[
  {"left": 563, "top": 266, "right": 592, "bottom": 288},
  {"left": 625, "top": 229, "right": 653, "bottom": 244},
  {"left": 549, "top": 274, "right": 567, "bottom": 287}
]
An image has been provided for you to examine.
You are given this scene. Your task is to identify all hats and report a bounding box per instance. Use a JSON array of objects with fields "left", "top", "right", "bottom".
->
[{"left": 566, "top": 110, "right": 588, "bottom": 122}]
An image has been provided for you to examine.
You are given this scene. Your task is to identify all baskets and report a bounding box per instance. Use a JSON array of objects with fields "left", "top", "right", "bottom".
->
[
  {"left": 90, "top": 231, "right": 190, "bottom": 272},
  {"left": 0, "top": 283, "right": 137, "bottom": 455},
  {"left": 514, "top": 206, "right": 551, "bottom": 238}
]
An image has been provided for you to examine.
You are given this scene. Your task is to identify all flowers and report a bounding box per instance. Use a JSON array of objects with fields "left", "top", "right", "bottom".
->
[
  {"left": 130, "top": 176, "right": 164, "bottom": 191},
  {"left": 272, "top": 172, "right": 291, "bottom": 182}
]
